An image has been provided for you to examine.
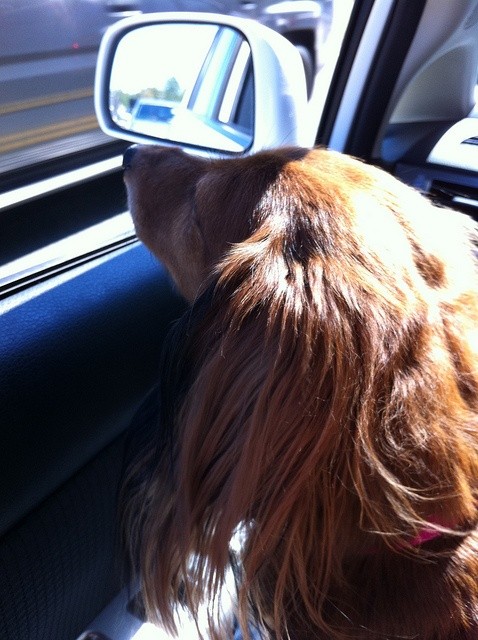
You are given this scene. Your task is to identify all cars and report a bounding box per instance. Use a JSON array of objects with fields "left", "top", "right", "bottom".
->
[{"left": 129, "top": 97, "right": 176, "bottom": 136}]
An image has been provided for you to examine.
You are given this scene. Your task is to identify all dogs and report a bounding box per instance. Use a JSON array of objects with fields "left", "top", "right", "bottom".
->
[{"left": 122, "top": 142, "right": 478, "bottom": 640}]
